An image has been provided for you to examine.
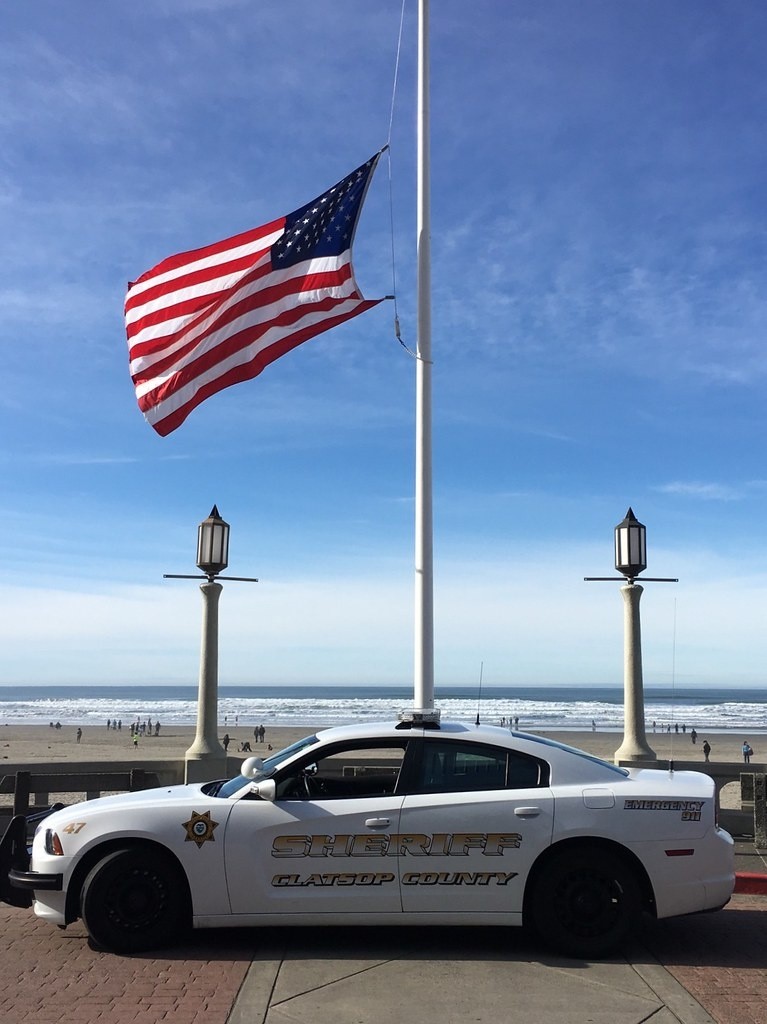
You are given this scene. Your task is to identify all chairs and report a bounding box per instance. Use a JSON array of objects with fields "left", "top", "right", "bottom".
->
[
  {"left": 422, "top": 749, "right": 446, "bottom": 792},
  {"left": 440, "top": 749, "right": 457, "bottom": 791}
]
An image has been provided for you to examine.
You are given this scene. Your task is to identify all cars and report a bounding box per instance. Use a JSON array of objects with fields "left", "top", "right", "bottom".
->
[{"left": 0, "top": 710, "right": 738, "bottom": 959}]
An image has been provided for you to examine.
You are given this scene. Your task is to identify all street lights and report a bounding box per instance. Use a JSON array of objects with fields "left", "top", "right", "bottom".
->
[
  {"left": 162, "top": 504, "right": 258, "bottom": 758},
  {"left": 584, "top": 506, "right": 679, "bottom": 762}
]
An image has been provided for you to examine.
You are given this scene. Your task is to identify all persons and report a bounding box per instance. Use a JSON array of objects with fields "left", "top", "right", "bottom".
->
[
  {"left": 107, "top": 719, "right": 122, "bottom": 730},
  {"left": 129, "top": 719, "right": 161, "bottom": 737},
  {"left": 742, "top": 741, "right": 751, "bottom": 763},
  {"left": 653, "top": 721, "right": 656, "bottom": 727},
  {"left": 703, "top": 740, "right": 711, "bottom": 762},
  {"left": 675, "top": 723, "right": 679, "bottom": 733},
  {"left": 691, "top": 729, "right": 697, "bottom": 744},
  {"left": 667, "top": 724, "right": 670, "bottom": 733},
  {"left": 254, "top": 725, "right": 265, "bottom": 743},
  {"left": 501, "top": 716, "right": 519, "bottom": 726},
  {"left": 133, "top": 731, "right": 139, "bottom": 749},
  {"left": 682, "top": 724, "right": 686, "bottom": 733},
  {"left": 77, "top": 728, "right": 82, "bottom": 743},
  {"left": 223, "top": 734, "right": 229, "bottom": 751},
  {"left": 238, "top": 741, "right": 252, "bottom": 752}
]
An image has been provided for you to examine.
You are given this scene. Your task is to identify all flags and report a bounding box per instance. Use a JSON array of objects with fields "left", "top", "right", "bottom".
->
[{"left": 125, "top": 144, "right": 392, "bottom": 437}]
